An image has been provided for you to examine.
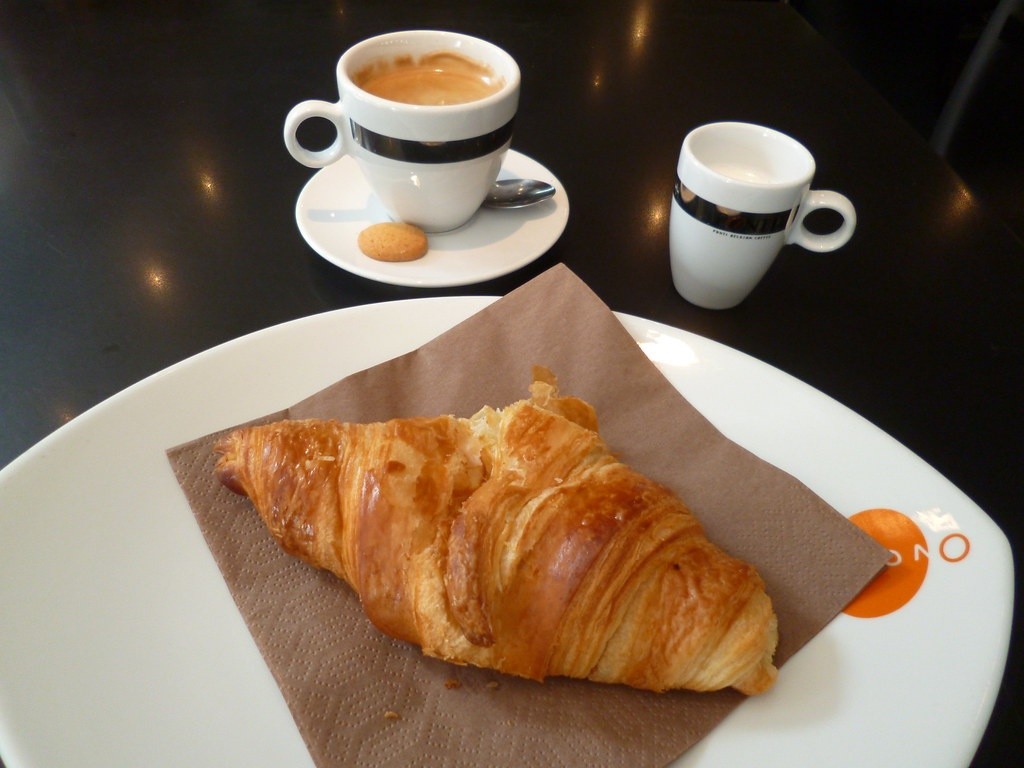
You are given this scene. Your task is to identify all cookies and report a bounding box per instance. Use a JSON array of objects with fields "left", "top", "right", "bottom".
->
[{"left": 358, "top": 222, "right": 429, "bottom": 262}]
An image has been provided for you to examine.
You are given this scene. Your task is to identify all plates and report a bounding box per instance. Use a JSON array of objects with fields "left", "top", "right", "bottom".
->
[
  {"left": 1, "top": 296, "right": 1015, "bottom": 768},
  {"left": 294, "top": 146, "right": 571, "bottom": 289}
]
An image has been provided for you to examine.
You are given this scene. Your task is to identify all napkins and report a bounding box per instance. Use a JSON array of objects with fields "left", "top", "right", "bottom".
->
[{"left": 167, "top": 261, "right": 894, "bottom": 768}]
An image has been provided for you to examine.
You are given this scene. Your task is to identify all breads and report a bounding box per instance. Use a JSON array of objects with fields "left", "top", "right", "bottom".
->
[{"left": 209, "top": 370, "right": 777, "bottom": 696}]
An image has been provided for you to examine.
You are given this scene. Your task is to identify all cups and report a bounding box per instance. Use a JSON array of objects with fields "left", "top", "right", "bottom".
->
[
  {"left": 670, "top": 121, "right": 858, "bottom": 310},
  {"left": 283, "top": 29, "right": 522, "bottom": 233}
]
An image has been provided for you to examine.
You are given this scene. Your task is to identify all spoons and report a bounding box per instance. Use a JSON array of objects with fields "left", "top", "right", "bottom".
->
[{"left": 481, "top": 178, "right": 557, "bottom": 211}]
